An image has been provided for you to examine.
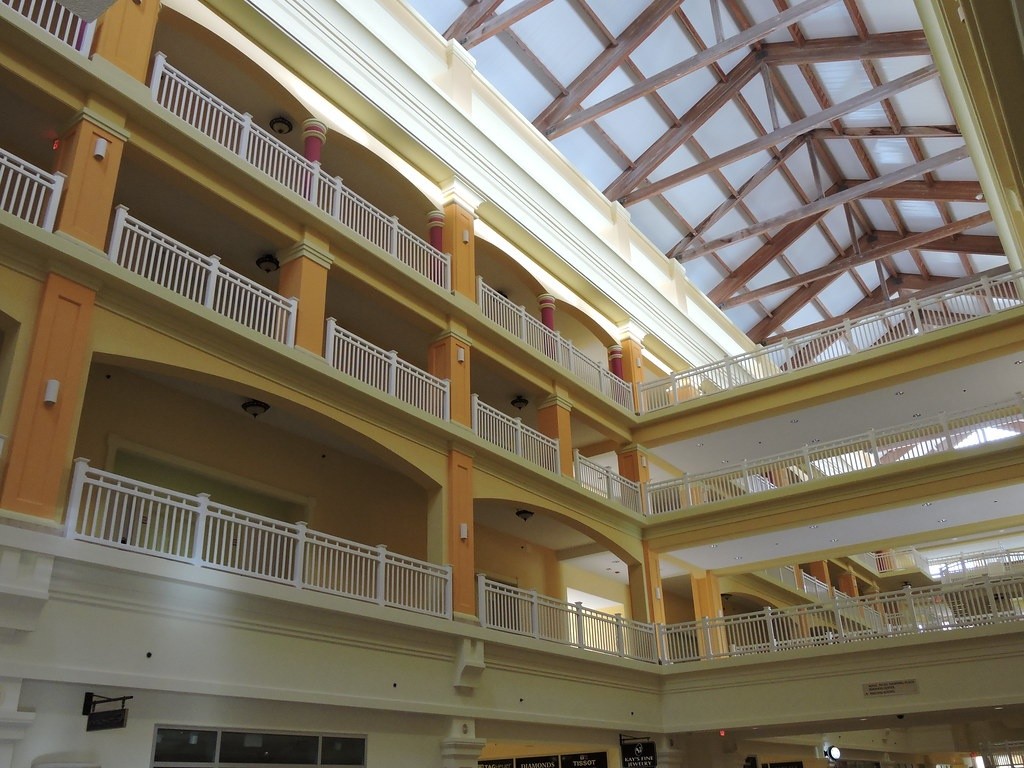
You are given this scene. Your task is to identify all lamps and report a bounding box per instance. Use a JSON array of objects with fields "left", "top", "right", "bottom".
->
[
  {"left": 511, "top": 395, "right": 529, "bottom": 411},
  {"left": 824, "top": 745, "right": 840, "bottom": 762},
  {"left": 902, "top": 582, "right": 912, "bottom": 589},
  {"left": 516, "top": 510, "right": 535, "bottom": 522},
  {"left": 269, "top": 117, "right": 293, "bottom": 135},
  {"left": 242, "top": 399, "right": 270, "bottom": 419},
  {"left": 255, "top": 254, "right": 280, "bottom": 274}
]
